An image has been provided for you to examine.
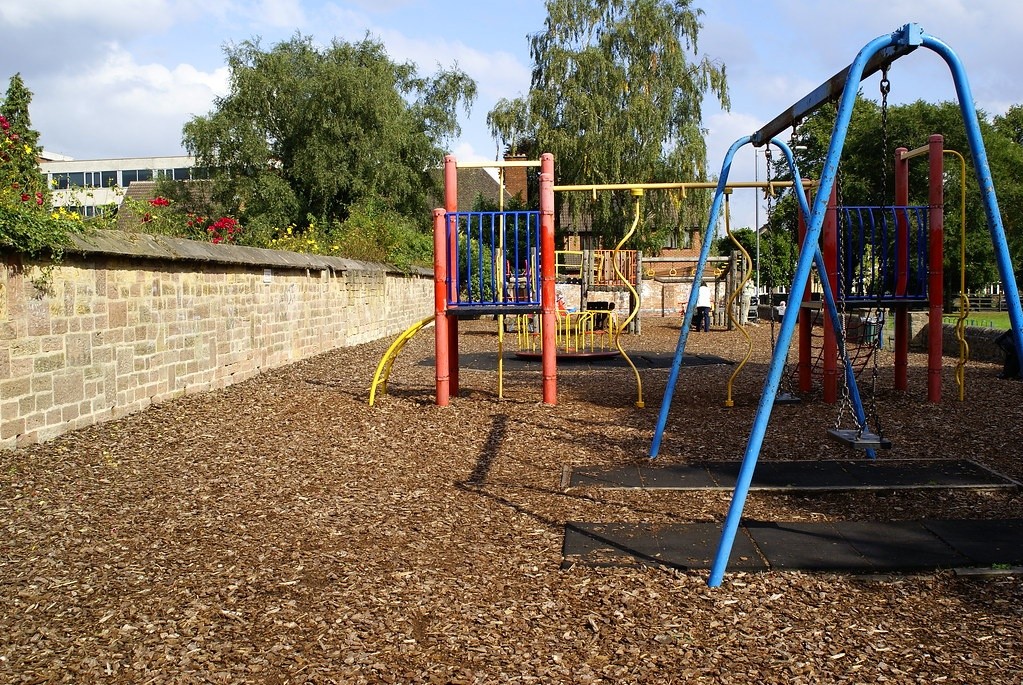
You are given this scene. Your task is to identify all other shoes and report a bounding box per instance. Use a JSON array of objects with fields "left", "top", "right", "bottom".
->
[
  {"left": 704, "top": 329, "right": 709, "bottom": 332},
  {"left": 696, "top": 328, "right": 700, "bottom": 332}
]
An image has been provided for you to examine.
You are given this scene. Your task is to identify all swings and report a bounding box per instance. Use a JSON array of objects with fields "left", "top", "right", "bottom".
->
[
  {"left": 763, "top": 132, "right": 804, "bottom": 406},
  {"left": 820, "top": 76, "right": 892, "bottom": 451}
]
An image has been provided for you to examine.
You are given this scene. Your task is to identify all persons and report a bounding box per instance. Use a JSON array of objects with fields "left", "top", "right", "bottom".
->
[
  {"left": 609, "top": 302, "right": 618, "bottom": 329},
  {"left": 695, "top": 281, "right": 710, "bottom": 332},
  {"left": 556, "top": 290, "right": 577, "bottom": 316},
  {"left": 777, "top": 301, "right": 786, "bottom": 323}
]
